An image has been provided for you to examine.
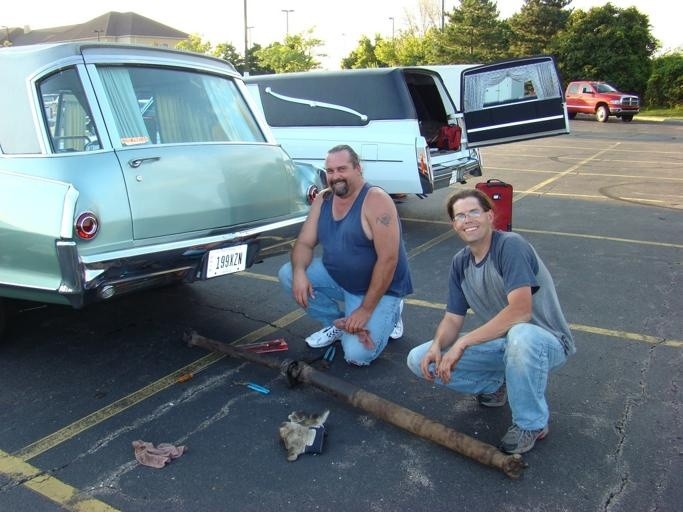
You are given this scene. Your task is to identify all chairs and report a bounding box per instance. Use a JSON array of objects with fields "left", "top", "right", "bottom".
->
[{"left": 143, "top": 118, "right": 158, "bottom": 144}]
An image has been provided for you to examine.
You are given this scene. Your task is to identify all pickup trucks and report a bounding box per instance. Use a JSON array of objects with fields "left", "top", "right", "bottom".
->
[{"left": 564, "top": 79, "right": 643, "bottom": 124}]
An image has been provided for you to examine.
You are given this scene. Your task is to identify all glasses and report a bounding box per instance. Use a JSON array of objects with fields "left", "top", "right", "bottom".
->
[{"left": 452, "top": 209, "right": 487, "bottom": 224}]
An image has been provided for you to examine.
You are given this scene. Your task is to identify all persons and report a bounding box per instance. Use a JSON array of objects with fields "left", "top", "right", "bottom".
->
[
  {"left": 406, "top": 189, "right": 575, "bottom": 455},
  {"left": 278, "top": 145, "right": 412, "bottom": 368}
]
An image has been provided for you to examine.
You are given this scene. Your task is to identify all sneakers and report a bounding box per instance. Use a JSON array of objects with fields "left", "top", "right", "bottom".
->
[
  {"left": 305, "top": 325, "right": 343, "bottom": 348},
  {"left": 500, "top": 423, "right": 548, "bottom": 454},
  {"left": 390, "top": 299, "right": 404, "bottom": 339},
  {"left": 478, "top": 377, "right": 507, "bottom": 406}
]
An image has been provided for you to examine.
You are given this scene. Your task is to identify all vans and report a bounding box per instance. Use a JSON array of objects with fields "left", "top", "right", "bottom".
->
[
  {"left": 243, "top": 57, "right": 572, "bottom": 205},
  {"left": 0, "top": 40, "right": 311, "bottom": 318}
]
